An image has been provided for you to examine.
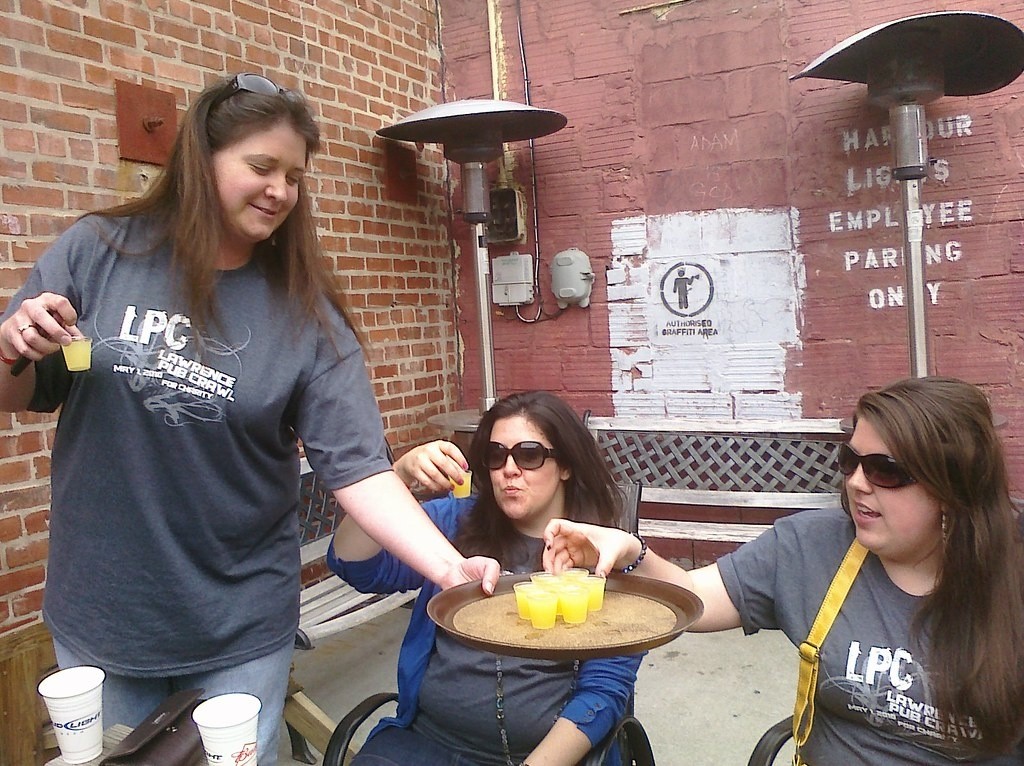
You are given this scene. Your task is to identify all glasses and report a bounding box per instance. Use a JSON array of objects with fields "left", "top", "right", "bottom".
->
[
  {"left": 211, "top": 72, "right": 305, "bottom": 111},
  {"left": 839, "top": 442, "right": 917, "bottom": 488},
  {"left": 482, "top": 441, "right": 555, "bottom": 470}
]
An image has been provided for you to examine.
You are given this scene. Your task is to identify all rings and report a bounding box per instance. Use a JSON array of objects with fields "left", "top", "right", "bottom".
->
[{"left": 17, "top": 323, "right": 40, "bottom": 336}]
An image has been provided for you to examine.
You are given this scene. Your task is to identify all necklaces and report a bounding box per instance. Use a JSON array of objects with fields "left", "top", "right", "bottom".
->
[{"left": 492, "top": 651, "right": 581, "bottom": 766}]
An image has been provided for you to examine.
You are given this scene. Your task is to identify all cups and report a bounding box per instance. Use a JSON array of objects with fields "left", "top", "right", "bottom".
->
[
  {"left": 513, "top": 568, "right": 606, "bottom": 629},
  {"left": 191, "top": 692, "right": 262, "bottom": 766},
  {"left": 37, "top": 665, "right": 107, "bottom": 765},
  {"left": 61, "top": 337, "right": 93, "bottom": 371},
  {"left": 449, "top": 470, "right": 472, "bottom": 499}
]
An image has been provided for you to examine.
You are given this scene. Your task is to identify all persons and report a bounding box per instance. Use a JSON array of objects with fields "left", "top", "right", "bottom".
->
[
  {"left": 1, "top": 75, "right": 507, "bottom": 764},
  {"left": 324, "top": 391, "right": 649, "bottom": 766},
  {"left": 542, "top": 378, "right": 1023, "bottom": 766}
]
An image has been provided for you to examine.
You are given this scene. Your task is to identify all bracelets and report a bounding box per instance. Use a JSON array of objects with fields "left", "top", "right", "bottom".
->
[
  {"left": 608, "top": 528, "right": 647, "bottom": 575},
  {"left": 0, "top": 350, "right": 18, "bottom": 366}
]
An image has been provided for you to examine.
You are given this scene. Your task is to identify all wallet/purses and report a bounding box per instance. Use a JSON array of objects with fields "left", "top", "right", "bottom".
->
[{"left": 100, "top": 688, "right": 207, "bottom": 766}]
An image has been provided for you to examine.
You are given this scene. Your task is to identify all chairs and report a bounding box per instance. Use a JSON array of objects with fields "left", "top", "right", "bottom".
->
[{"left": 321, "top": 480, "right": 656, "bottom": 766}]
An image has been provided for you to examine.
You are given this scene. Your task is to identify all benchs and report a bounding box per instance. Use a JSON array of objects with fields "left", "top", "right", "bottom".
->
[
  {"left": 579, "top": 415, "right": 855, "bottom": 546},
  {"left": 280, "top": 445, "right": 422, "bottom": 765}
]
